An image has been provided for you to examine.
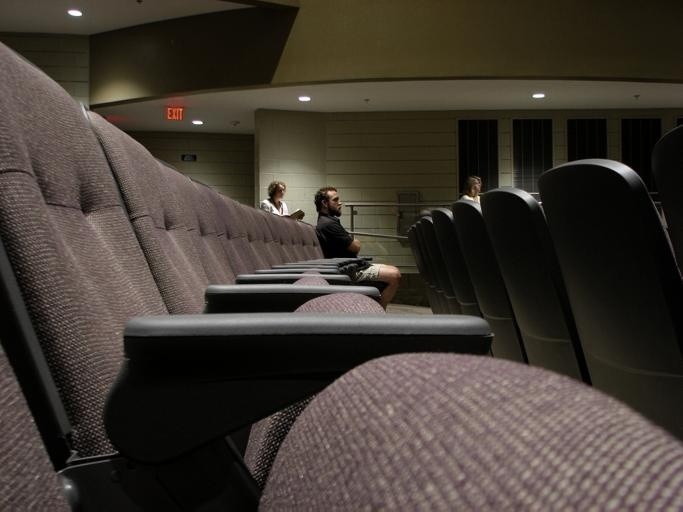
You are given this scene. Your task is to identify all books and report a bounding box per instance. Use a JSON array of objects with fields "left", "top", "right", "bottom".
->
[{"left": 290, "top": 209, "right": 305, "bottom": 221}]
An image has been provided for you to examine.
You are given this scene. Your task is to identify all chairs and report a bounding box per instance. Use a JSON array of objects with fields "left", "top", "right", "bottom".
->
[{"left": 1, "top": 41, "right": 682, "bottom": 512}]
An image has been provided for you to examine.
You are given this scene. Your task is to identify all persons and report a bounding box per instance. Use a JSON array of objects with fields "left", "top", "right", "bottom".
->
[
  {"left": 460, "top": 175, "right": 483, "bottom": 217},
  {"left": 311, "top": 185, "right": 403, "bottom": 313},
  {"left": 257, "top": 179, "right": 296, "bottom": 222}
]
